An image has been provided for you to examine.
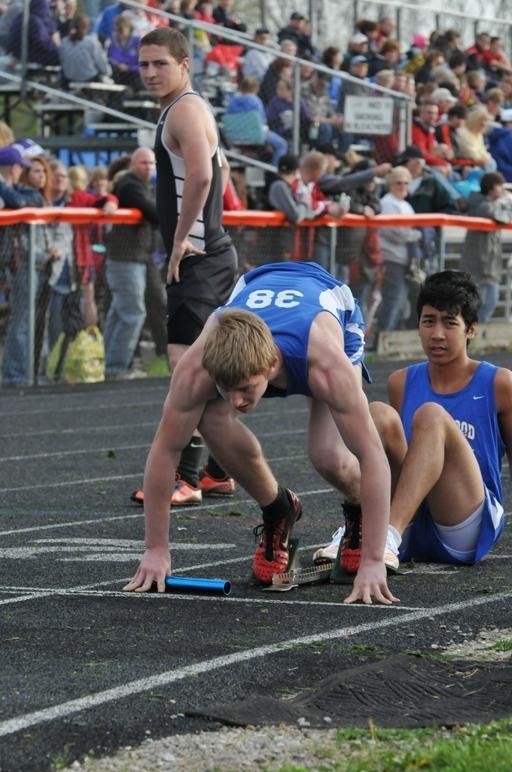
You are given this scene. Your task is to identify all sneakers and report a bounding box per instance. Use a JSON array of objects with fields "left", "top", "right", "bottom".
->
[
  {"left": 336, "top": 499, "right": 367, "bottom": 577},
  {"left": 129, "top": 475, "right": 205, "bottom": 508},
  {"left": 379, "top": 536, "right": 401, "bottom": 573},
  {"left": 197, "top": 462, "right": 237, "bottom": 499},
  {"left": 312, "top": 524, "right": 347, "bottom": 567},
  {"left": 250, "top": 487, "right": 306, "bottom": 586}
]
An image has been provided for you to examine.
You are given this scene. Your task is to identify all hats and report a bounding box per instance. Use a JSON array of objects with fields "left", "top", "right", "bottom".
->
[
  {"left": 349, "top": 55, "right": 368, "bottom": 66},
  {"left": 289, "top": 12, "right": 305, "bottom": 23},
  {"left": 1, "top": 147, "right": 33, "bottom": 171},
  {"left": 429, "top": 87, "right": 458, "bottom": 105},
  {"left": 392, "top": 144, "right": 424, "bottom": 161}
]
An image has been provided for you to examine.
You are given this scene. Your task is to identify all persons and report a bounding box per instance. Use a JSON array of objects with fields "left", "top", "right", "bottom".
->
[
  {"left": 122, "top": 261, "right": 400, "bottom": 606},
  {"left": 311, "top": 270, "right": 511, "bottom": 578},
  {"left": 130, "top": 28, "right": 242, "bottom": 507}
]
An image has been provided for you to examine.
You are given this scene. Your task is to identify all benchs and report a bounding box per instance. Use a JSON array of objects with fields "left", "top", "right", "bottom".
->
[{"left": 1, "top": 12, "right": 512, "bottom": 215}]
